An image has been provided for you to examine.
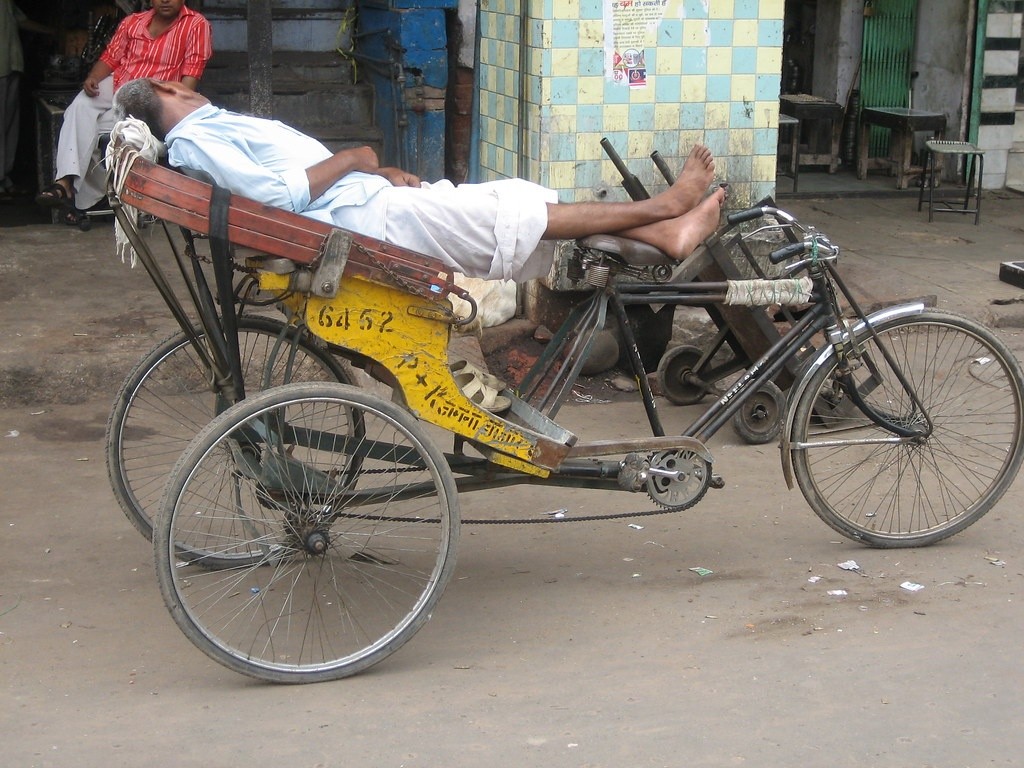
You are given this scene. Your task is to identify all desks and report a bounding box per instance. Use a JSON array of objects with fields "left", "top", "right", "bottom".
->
[
  {"left": 860, "top": 105, "right": 947, "bottom": 189},
  {"left": 778, "top": 92, "right": 844, "bottom": 174}
]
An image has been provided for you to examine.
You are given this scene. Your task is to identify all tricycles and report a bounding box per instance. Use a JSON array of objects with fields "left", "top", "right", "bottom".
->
[{"left": 102, "top": 137, "right": 1024, "bottom": 684}]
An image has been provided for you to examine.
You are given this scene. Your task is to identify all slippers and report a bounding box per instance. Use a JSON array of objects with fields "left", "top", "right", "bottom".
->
[
  {"left": 36, "top": 184, "right": 75, "bottom": 207},
  {"left": 454, "top": 372, "right": 511, "bottom": 412},
  {"left": 449, "top": 360, "right": 506, "bottom": 390}
]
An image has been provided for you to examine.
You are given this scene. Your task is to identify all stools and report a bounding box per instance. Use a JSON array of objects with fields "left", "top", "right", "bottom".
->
[
  {"left": 778, "top": 113, "right": 799, "bottom": 192},
  {"left": 917, "top": 139, "right": 985, "bottom": 225}
]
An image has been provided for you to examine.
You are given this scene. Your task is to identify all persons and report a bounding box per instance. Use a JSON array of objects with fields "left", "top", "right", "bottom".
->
[
  {"left": 113, "top": 76, "right": 725, "bottom": 283},
  {"left": 34, "top": 0, "right": 215, "bottom": 224},
  {"left": 0, "top": 0, "right": 57, "bottom": 203}
]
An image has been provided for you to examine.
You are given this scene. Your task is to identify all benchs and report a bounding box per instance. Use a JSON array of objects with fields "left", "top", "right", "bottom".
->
[{"left": 106, "top": 135, "right": 493, "bottom": 414}]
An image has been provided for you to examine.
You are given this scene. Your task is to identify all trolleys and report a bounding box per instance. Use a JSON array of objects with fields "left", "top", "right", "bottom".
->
[{"left": 597, "top": 135, "right": 883, "bottom": 445}]
